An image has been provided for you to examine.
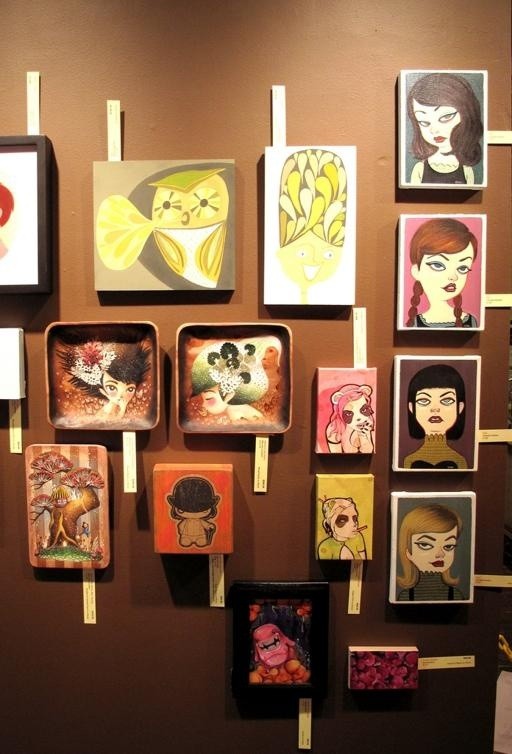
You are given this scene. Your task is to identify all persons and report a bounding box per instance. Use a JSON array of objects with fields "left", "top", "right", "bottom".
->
[
  {"left": 397, "top": 504, "right": 465, "bottom": 600},
  {"left": 319, "top": 384, "right": 374, "bottom": 454},
  {"left": 402, "top": 363, "right": 468, "bottom": 468},
  {"left": 406, "top": 219, "right": 477, "bottom": 328},
  {"left": 407, "top": 73, "right": 483, "bottom": 184},
  {"left": 63, "top": 346, "right": 150, "bottom": 420},
  {"left": 317, "top": 498, "right": 367, "bottom": 560}
]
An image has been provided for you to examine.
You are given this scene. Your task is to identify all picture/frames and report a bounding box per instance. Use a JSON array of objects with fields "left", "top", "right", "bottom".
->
[
  {"left": 393, "top": 210, "right": 489, "bottom": 334},
  {"left": 396, "top": 68, "right": 490, "bottom": 192},
  {"left": 390, "top": 352, "right": 482, "bottom": 473},
  {"left": 388, "top": 489, "right": 476, "bottom": 608},
  {"left": 225, "top": 576, "right": 333, "bottom": 700},
  {"left": 0, "top": 132, "right": 55, "bottom": 298}
]
